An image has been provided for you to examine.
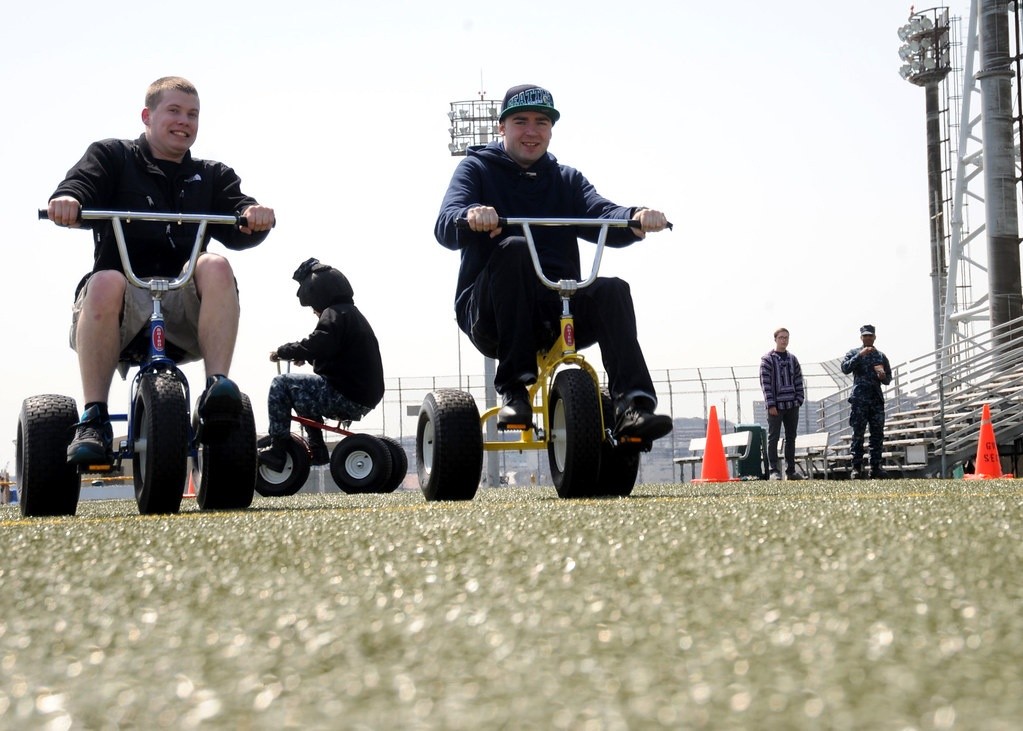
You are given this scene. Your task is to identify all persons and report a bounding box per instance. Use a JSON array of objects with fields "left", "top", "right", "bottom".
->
[
  {"left": 49, "top": 76, "right": 276, "bottom": 465},
  {"left": 256, "top": 257, "right": 385, "bottom": 472},
  {"left": 759, "top": 328, "right": 805, "bottom": 480},
  {"left": 435, "top": 84, "right": 673, "bottom": 442},
  {"left": 841, "top": 324, "right": 892, "bottom": 479}
]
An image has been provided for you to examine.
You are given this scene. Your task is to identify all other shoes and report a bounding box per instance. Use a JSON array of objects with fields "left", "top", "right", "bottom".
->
[
  {"left": 312, "top": 445, "right": 329, "bottom": 466},
  {"left": 257, "top": 445, "right": 287, "bottom": 473},
  {"left": 851, "top": 469, "right": 866, "bottom": 479},
  {"left": 870, "top": 470, "right": 890, "bottom": 478},
  {"left": 772, "top": 472, "right": 781, "bottom": 479},
  {"left": 502, "top": 387, "right": 531, "bottom": 421},
  {"left": 788, "top": 473, "right": 803, "bottom": 480},
  {"left": 614, "top": 408, "right": 672, "bottom": 440}
]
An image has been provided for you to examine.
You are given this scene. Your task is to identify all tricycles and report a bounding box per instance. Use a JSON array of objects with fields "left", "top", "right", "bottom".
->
[
  {"left": 256, "top": 354, "right": 409, "bottom": 494},
  {"left": 15, "top": 208, "right": 277, "bottom": 517},
  {"left": 414, "top": 220, "right": 673, "bottom": 501}
]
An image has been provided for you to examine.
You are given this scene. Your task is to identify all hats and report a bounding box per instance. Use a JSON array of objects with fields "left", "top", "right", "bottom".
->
[
  {"left": 860, "top": 325, "right": 875, "bottom": 335},
  {"left": 499, "top": 85, "right": 561, "bottom": 123}
]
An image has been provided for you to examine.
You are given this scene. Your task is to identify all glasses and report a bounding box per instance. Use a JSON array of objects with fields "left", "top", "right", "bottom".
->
[{"left": 778, "top": 336, "right": 788, "bottom": 340}]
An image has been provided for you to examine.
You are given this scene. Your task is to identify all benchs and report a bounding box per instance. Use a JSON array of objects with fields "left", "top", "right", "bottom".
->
[
  {"left": 761, "top": 432, "right": 830, "bottom": 480},
  {"left": 673, "top": 431, "right": 753, "bottom": 482}
]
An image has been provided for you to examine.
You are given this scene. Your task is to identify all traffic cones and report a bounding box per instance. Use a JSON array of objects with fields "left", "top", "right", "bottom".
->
[
  {"left": 962, "top": 402, "right": 1013, "bottom": 479},
  {"left": 690, "top": 405, "right": 742, "bottom": 482}
]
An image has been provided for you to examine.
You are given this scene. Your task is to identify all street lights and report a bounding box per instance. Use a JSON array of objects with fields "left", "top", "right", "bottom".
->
[
  {"left": 447, "top": 95, "right": 505, "bottom": 489},
  {"left": 897, "top": 5, "right": 953, "bottom": 393}
]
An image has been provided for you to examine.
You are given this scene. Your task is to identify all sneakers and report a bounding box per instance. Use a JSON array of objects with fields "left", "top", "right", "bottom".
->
[
  {"left": 197, "top": 380, "right": 241, "bottom": 444},
  {"left": 67, "top": 405, "right": 113, "bottom": 466}
]
